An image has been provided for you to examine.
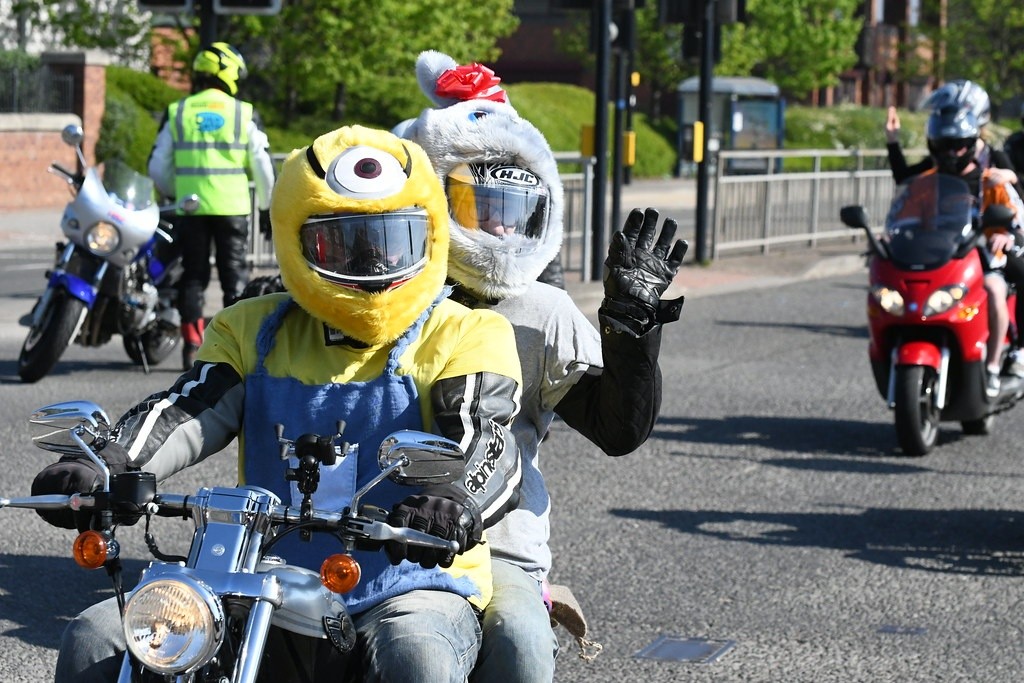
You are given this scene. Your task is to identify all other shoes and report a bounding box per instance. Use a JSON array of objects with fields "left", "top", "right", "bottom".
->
[
  {"left": 1004, "top": 348, "right": 1024, "bottom": 378},
  {"left": 183, "top": 342, "right": 204, "bottom": 371},
  {"left": 984, "top": 365, "right": 1001, "bottom": 397}
]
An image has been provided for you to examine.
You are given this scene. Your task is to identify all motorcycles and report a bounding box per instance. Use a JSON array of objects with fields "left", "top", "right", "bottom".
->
[
  {"left": 14, "top": 123, "right": 200, "bottom": 385},
  {"left": 0, "top": 399, "right": 607, "bottom": 683},
  {"left": 838, "top": 173, "right": 1024, "bottom": 457}
]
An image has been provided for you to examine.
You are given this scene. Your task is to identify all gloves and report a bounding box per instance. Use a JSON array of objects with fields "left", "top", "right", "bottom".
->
[
  {"left": 601, "top": 208, "right": 688, "bottom": 321},
  {"left": 384, "top": 484, "right": 483, "bottom": 569},
  {"left": 31, "top": 441, "right": 137, "bottom": 530},
  {"left": 259, "top": 210, "right": 273, "bottom": 241}
]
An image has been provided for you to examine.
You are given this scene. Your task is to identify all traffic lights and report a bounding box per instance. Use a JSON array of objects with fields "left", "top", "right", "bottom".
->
[{"left": 679, "top": 121, "right": 705, "bottom": 164}]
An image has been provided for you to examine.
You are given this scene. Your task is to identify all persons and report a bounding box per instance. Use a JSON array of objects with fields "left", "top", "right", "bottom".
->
[
  {"left": 31, "top": 124, "right": 524, "bottom": 683},
  {"left": 241, "top": 50, "right": 690, "bottom": 683},
  {"left": 886, "top": 82, "right": 1024, "bottom": 400},
  {"left": 147, "top": 42, "right": 274, "bottom": 370}
]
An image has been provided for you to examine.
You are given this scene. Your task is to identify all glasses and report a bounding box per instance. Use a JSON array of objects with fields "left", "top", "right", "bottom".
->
[
  {"left": 930, "top": 139, "right": 975, "bottom": 155},
  {"left": 453, "top": 196, "right": 533, "bottom": 227}
]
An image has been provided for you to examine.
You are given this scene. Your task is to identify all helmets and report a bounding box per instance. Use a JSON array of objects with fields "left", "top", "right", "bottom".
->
[
  {"left": 444, "top": 161, "right": 551, "bottom": 242},
  {"left": 297, "top": 204, "right": 432, "bottom": 296},
  {"left": 926, "top": 104, "right": 981, "bottom": 176},
  {"left": 191, "top": 42, "right": 249, "bottom": 96},
  {"left": 921, "top": 79, "right": 991, "bottom": 126}
]
